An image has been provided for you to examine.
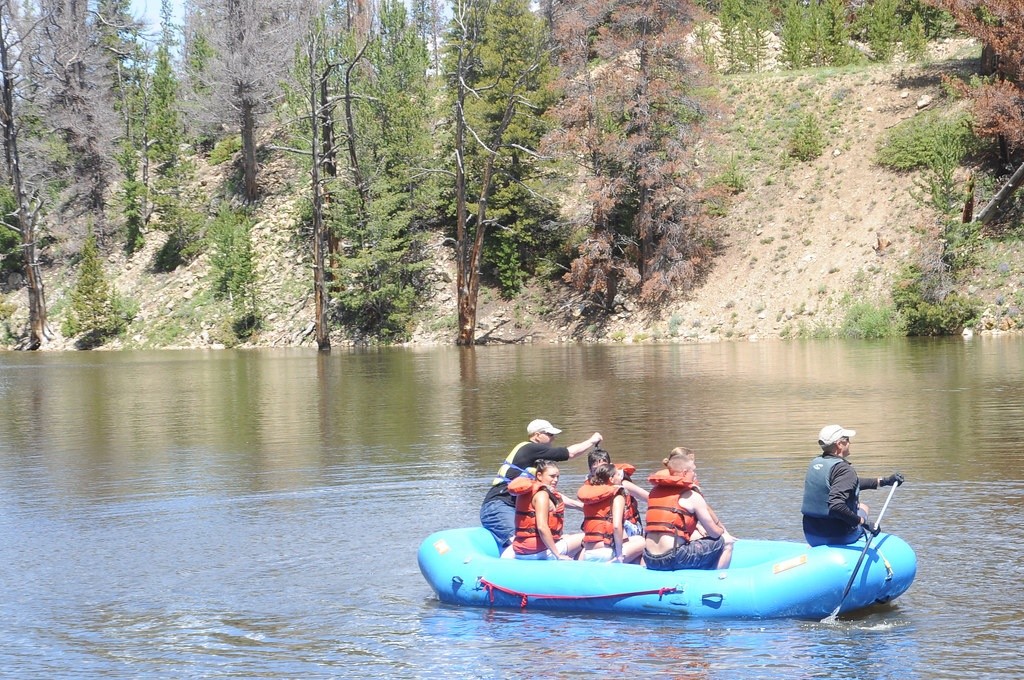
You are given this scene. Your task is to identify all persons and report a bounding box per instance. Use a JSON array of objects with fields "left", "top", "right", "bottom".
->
[
  {"left": 480, "top": 418, "right": 604, "bottom": 549},
  {"left": 579, "top": 463, "right": 645, "bottom": 566},
  {"left": 800, "top": 424, "right": 904, "bottom": 547},
  {"left": 662, "top": 446, "right": 738, "bottom": 570},
  {"left": 641, "top": 455, "right": 725, "bottom": 570},
  {"left": 588, "top": 449, "right": 650, "bottom": 538},
  {"left": 512, "top": 459, "right": 586, "bottom": 560}
]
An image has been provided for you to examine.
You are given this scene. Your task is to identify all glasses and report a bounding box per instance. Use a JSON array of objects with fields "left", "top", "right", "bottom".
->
[
  {"left": 833, "top": 438, "right": 849, "bottom": 443},
  {"left": 542, "top": 432, "right": 554, "bottom": 436}
]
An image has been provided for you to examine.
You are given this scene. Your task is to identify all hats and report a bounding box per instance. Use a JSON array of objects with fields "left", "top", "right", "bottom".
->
[
  {"left": 526, "top": 419, "right": 562, "bottom": 434},
  {"left": 818, "top": 425, "right": 856, "bottom": 447}
]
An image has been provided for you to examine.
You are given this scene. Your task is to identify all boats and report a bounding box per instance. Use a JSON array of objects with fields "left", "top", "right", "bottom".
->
[{"left": 416, "top": 526, "right": 916, "bottom": 619}]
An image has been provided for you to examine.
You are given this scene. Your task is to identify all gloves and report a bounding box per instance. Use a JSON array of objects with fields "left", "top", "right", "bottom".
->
[
  {"left": 884, "top": 473, "right": 904, "bottom": 487},
  {"left": 860, "top": 518, "right": 881, "bottom": 537}
]
